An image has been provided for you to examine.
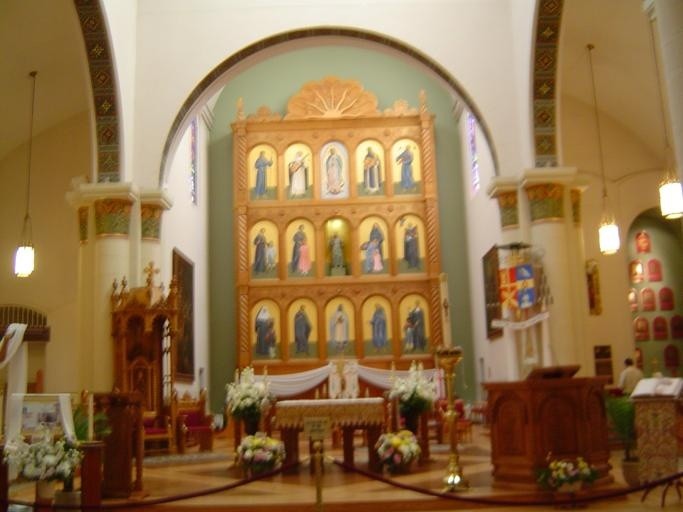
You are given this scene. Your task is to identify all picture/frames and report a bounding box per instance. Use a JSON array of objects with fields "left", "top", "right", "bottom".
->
[
  {"left": 483, "top": 245, "right": 503, "bottom": 341},
  {"left": 172, "top": 246, "right": 195, "bottom": 384}
]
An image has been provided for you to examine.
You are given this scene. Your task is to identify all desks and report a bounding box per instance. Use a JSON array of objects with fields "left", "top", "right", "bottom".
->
[{"left": 275, "top": 397, "right": 387, "bottom": 471}]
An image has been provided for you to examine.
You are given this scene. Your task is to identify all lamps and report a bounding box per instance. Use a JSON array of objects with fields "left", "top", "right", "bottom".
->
[
  {"left": 648, "top": 21, "right": 683, "bottom": 221},
  {"left": 587, "top": 43, "right": 621, "bottom": 254},
  {"left": 14, "top": 71, "right": 39, "bottom": 277}
]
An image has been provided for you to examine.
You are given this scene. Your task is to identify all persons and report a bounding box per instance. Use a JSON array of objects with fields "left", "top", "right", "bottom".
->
[
  {"left": 293, "top": 302, "right": 313, "bottom": 357},
  {"left": 327, "top": 229, "right": 348, "bottom": 276},
  {"left": 251, "top": 226, "right": 276, "bottom": 274},
  {"left": 360, "top": 221, "right": 389, "bottom": 274},
  {"left": 403, "top": 299, "right": 429, "bottom": 353},
  {"left": 370, "top": 301, "right": 388, "bottom": 353},
  {"left": 402, "top": 222, "right": 427, "bottom": 271},
  {"left": 324, "top": 146, "right": 346, "bottom": 194},
  {"left": 615, "top": 358, "right": 647, "bottom": 399},
  {"left": 248, "top": 146, "right": 274, "bottom": 199},
  {"left": 290, "top": 222, "right": 313, "bottom": 275},
  {"left": 397, "top": 145, "right": 418, "bottom": 191},
  {"left": 328, "top": 303, "right": 351, "bottom": 348},
  {"left": 283, "top": 147, "right": 312, "bottom": 200},
  {"left": 361, "top": 146, "right": 383, "bottom": 192},
  {"left": 254, "top": 304, "right": 279, "bottom": 359}
]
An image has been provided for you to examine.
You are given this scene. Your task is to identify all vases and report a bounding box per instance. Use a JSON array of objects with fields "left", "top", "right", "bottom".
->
[
  {"left": 74, "top": 475, "right": 81, "bottom": 489},
  {"left": 35, "top": 480, "right": 64, "bottom": 504},
  {"left": 54, "top": 490, "right": 83, "bottom": 511}
]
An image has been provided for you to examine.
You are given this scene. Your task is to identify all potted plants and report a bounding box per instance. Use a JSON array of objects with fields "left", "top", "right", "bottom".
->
[
  {"left": 603, "top": 395, "right": 640, "bottom": 488},
  {"left": 73, "top": 407, "right": 112, "bottom": 508}
]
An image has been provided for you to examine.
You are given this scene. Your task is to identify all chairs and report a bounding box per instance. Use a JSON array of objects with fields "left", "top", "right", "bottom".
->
[
  {"left": 438, "top": 398, "right": 473, "bottom": 443},
  {"left": 138, "top": 409, "right": 178, "bottom": 456},
  {"left": 174, "top": 389, "right": 215, "bottom": 453}
]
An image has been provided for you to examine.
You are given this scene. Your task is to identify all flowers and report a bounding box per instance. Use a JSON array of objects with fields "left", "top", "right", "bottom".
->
[
  {"left": 234, "top": 431, "right": 287, "bottom": 481},
  {"left": 532, "top": 452, "right": 613, "bottom": 491},
  {"left": 224, "top": 364, "right": 277, "bottom": 430},
  {"left": 2, "top": 425, "right": 72, "bottom": 481},
  {"left": 387, "top": 361, "right": 436, "bottom": 432},
  {"left": 69, "top": 448, "right": 85, "bottom": 474},
  {"left": 374, "top": 430, "right": 422, "bottom": 476}
]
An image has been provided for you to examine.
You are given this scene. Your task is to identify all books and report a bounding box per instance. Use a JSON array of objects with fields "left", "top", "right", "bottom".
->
[{"left": 628, "top": 374, "right": 683, "bottom": 401}]
